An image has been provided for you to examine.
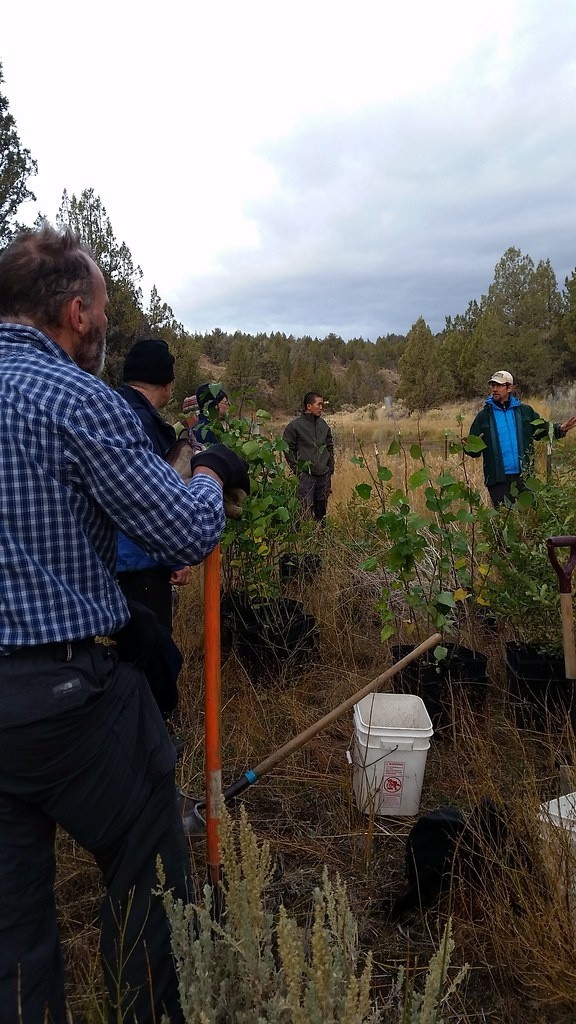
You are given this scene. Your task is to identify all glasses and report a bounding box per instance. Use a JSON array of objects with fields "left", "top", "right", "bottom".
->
[{"left": 489, "top": 383, "right": 506, "bottom": 390}]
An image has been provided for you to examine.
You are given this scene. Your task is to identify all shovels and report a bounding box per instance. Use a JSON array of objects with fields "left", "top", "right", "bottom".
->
[
  {"left": 546, "top": 536, "right": 575, "bottom": 679},
  {"left": 173, "top": 630, "right": 445, "bottom": 848},
  {"left": 202, "top": 540, "right": 228, "bottom": 940}
]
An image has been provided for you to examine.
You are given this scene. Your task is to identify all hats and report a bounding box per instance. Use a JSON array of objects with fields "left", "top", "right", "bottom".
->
[
  {"left": 196, "top": 383, "right": 228, "bottom": 413},
  {"left": 122, "top": 338, "right": 176, "bottom": 386},
  {"left": 487, "top": 370, "right": 514, "bottom": 384},
  {"left": 182, "top": 396, "right": 200, "bottom": 414}
]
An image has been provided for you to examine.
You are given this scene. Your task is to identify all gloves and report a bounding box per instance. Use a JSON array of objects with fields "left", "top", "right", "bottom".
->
[{"left": 190, "top": 444, "right": 251, "bottom": 496}]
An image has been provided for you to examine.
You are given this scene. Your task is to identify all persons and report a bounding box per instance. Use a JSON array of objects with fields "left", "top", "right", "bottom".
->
[
  {"left": 461, "top": 371, "right": 576, "bottom": 511},
  {"left": 282, "top": 392, "right": 334, "bottom": 578},
  {"left": 0, "top": 235, "right": 248, "bottom": 1024}
]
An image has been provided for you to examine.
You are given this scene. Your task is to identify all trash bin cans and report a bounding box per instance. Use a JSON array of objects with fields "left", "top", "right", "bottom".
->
[
  {"left": 536, "top": 791, "right": 576, "bottom": 899},
  {"left": 349, "top": 690, "right": 434, "bottom": 818},
  {"left": 506, "top": 640, "right": 576, "bottom": 737},
  {"left": 390, "top": 640, "right": 488, "bottom": 734}
]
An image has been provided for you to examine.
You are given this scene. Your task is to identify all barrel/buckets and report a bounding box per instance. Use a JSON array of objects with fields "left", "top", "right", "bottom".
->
[
  {"left": 537, "top": 794, "right": 576, "bottom": 899},
  {"left": 346, "top": 692, "right": 434, "bottom": 817}
]
